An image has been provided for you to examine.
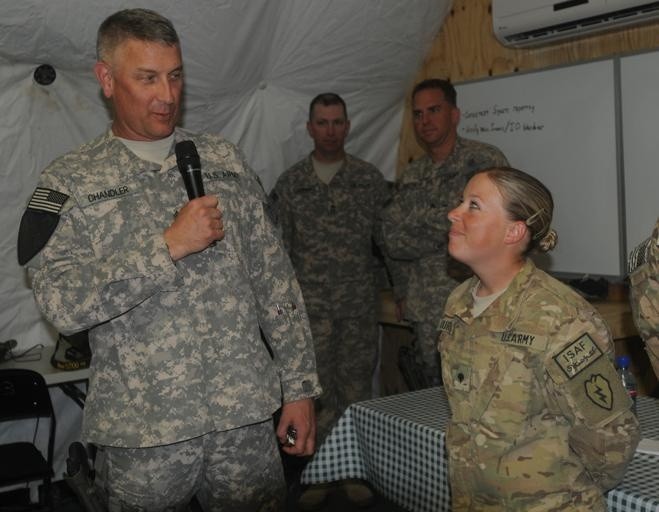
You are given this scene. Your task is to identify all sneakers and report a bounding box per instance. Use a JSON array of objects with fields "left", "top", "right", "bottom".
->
[
  {"left": 341, "top": 479, "right": 372, "bottom": 505},
  {"left": 299, "top": 483, "right": 329, "bottom": 508}
]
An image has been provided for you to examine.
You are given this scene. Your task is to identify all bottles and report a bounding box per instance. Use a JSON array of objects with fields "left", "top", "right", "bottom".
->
[{"left": 616, "top": 356, "right": 637, "bottom": 417}]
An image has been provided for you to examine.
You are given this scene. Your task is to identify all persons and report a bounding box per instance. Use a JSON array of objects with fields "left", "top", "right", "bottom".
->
[
  {"left": 260, "top": 93, "right": 394, "bottom": 508},
  {"left": 625, "top": 218, "right": 659, "bottom": 379},
  {"left": 16, "top": 9, "right": 323, "bottom": 512},
  {"left": 371, "top": 79, "right": 514, "bottom": 386},
  {"left": 435, "top": 165, "right": 641, "bottom": 511}
]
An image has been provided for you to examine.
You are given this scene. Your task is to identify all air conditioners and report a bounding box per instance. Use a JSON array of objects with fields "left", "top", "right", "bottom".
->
[{"left": 491, "top": 0, "right": 659, "bottom": 50}]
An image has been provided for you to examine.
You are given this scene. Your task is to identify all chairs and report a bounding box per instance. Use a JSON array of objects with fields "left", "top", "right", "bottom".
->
[{"left": 0, "top": 368, "right": 56, "bottom": 510}]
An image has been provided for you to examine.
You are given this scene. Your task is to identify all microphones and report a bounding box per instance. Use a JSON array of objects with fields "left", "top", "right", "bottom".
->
[{"left": 174, "top": 140, "right": 216, "bottom": 247}]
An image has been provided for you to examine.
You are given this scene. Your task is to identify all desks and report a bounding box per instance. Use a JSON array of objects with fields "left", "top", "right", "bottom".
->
[{"left": 348, "top": 385, "right": 657, "bottom": 511}]
